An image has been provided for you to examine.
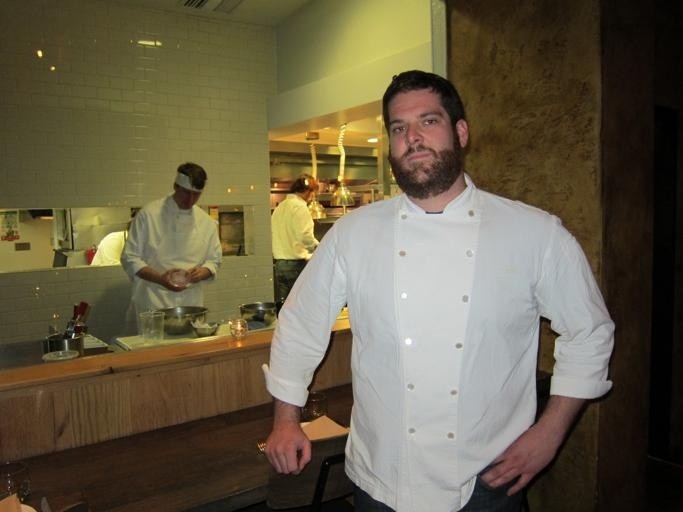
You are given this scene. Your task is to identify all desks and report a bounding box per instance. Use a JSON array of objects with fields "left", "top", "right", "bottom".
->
[{"left": 0, "top": 318, "right": 354, "bottom": 512}]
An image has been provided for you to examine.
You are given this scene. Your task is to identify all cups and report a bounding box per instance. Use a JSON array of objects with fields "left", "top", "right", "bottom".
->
[
  {"left": 228, "top": 318, "right": 249, "bottom": 341},
  {"left": 139, "top": 309, "right": 166, "bottom": 348}
]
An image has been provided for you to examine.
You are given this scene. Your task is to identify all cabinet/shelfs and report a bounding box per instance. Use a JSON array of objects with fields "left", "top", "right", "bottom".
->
[{"left": 53, "top": 208, "right": 131, "bottom": 251}]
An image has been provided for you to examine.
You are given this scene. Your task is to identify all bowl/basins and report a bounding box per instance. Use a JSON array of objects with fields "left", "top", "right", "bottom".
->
[
  {"left": 239, "top": 301, "right": 277, "bottom": 327},
  {"left": 157, "top": 305, "right": 210, "bottom": 336},
  {"left": 192, "top": 322, "right": 220, "bottom": 337}
]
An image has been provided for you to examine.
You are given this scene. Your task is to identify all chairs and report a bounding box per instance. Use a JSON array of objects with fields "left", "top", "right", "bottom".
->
[{"left": 267, "top": 432, "right": 356, "bottom": 512}]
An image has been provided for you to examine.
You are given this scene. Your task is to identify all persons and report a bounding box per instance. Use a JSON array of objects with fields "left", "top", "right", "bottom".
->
[
  {"left": 270, "top": 174, "right": 320, "bottom": 312},
  {"left": 121, "top": 162, "right": 223, "bottom": 327},
  {"left": 90, "top": 210, "right": 138, "bottom": 266},
  {"left": 264, "top": 71, "right": 615, "bottom": 511}
]
